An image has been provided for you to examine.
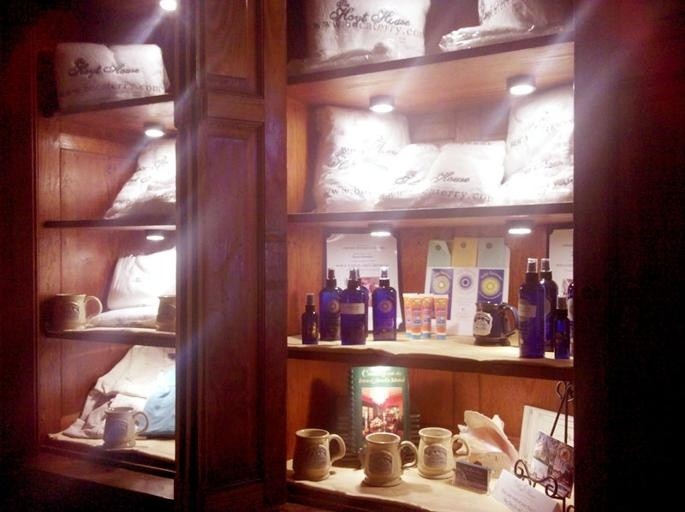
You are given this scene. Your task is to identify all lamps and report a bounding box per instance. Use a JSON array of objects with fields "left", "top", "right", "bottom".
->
[
  {"left": 369, "top": 94, "right": 394, "bottom": 112},
  {"left": 507, "top": 221, "right": 532, "bottom": 235},
  {"left": 143, "top": 123, "right": 164, "bottom": 138},
  {"left": 145, "top": 230, "right": 165, "bottom": 241},
  {"left": 368, "top": 223, "right": 393, "bottom": 237},
  {"left": 508, "top": 74, "right": 535, "bottom": 96}
]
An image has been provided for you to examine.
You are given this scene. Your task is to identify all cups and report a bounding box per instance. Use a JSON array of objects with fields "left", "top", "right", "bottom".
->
[
  {"left": 418, "top": 426, "right": 471, "bottom": 481},
  {"left": 54, "top": 293, "right": 104, "bottom": 330},
  {"left": 362, "top": 432, "right": 419, "bottom": 488},
  {"left": 155, "top": 295, "right": 176, "bottom": 332},
  {"left": 472, "top": 302, "right": 519, "bottom": 343},
  {"left": 103, "top": 406, "right": 149, "bottom": 450},
  {"left": 292, "top": 429, "right": 346, "bottom": 482}
]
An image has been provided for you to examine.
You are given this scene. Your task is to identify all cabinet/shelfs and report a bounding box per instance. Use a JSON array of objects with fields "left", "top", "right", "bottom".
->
[
  {"left": 258, "top": 0, "right": 685, "bottom": 512},
  {"left": 0, "top": 0, "right": 257, "bottom": 512}
]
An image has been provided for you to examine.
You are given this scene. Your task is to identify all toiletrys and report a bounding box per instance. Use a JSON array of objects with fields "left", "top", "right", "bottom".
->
[{"left": 300, "top": 256, "right": 574, "bottom": 360}]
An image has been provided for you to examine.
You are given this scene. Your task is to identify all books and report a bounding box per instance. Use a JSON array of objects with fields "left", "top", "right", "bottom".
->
[{"left": 348, "top": 363, "right": 412, "bottom": 464}]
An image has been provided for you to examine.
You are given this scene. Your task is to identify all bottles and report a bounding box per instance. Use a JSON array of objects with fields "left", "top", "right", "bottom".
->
[
  {"left": 301, "top": 292, "right": 319, "bottom": 344},
  {"left": 539, "top": 258, "right": 558, "bottom": 351},
  {"left": 340, "top": 268, "right": 366, "bottom": 345},
  {"left": 371, "top": 266, "right": 397, "bottom": 342},
  {"left": 319, "top": 266, "right": 343, "bottom": 340},
  {"left": 353, "top": 267, "right": 369, "bottom": 338},
  {"left": 553, "top": 295, "right": 571, "bottom": 360},
  {"left": 518, "top": 257, "right": 545, "bottom": 358}
]
